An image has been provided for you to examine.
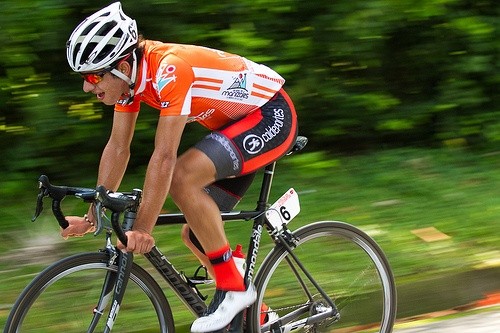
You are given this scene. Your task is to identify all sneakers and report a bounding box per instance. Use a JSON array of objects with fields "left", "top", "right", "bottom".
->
[
  {"left": 260, "top": 307, "right": 280, "bottom": 333},
  {"left": 186, "top": 280, "right": 257, "bottom": 332}
]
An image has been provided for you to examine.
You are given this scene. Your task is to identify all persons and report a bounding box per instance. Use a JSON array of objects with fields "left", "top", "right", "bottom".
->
[{"left": 57, "top": 2, "right": 297, "bottom": 333}]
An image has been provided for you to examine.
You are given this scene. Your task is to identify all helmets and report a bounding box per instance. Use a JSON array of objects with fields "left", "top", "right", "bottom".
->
[{"left": 66, "top": 1, "right": 142, "bottom": 76}]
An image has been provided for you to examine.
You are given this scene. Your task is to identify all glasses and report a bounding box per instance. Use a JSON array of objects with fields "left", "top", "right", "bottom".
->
[{"left": 78, "top": 54, "right": 132, "bottom": 86}]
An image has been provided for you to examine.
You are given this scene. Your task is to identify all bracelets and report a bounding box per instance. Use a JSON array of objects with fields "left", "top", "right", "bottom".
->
[{"left": 84, "top": 213, "right": 96, "bottom": 227}]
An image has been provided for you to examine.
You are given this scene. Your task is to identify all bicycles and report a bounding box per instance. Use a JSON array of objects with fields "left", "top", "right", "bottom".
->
[{"left": 4, "top": 135, "right": 398, "bottom": 333}]
